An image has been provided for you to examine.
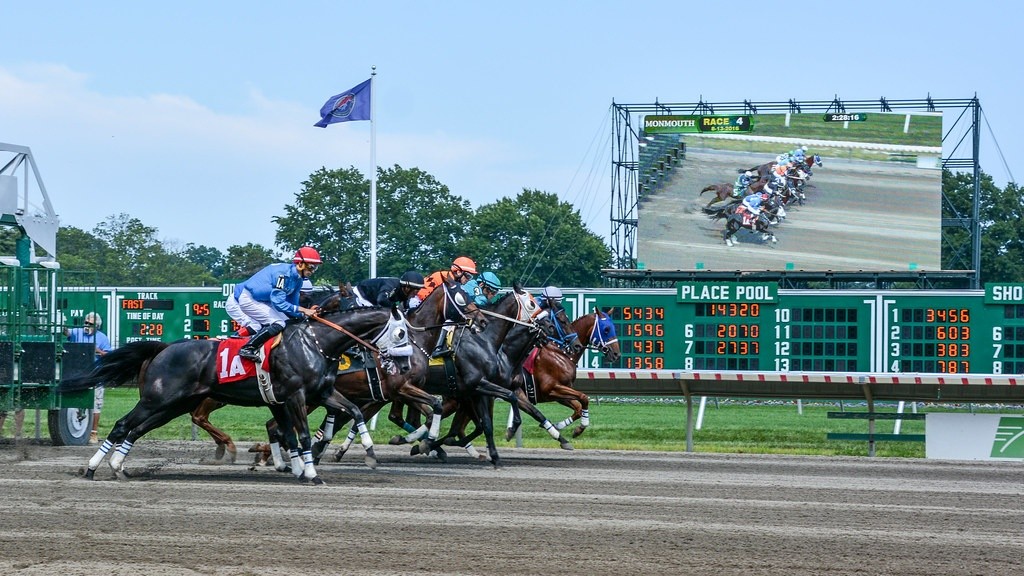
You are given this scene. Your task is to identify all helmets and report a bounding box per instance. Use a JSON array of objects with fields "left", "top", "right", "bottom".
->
[
  {"left": 292, "top": 247, "right": 324, "bottom": 264},
  {"left": 400, "top": 271, "right": 428, "bottom": 290},
  {"left": 540, "top": 285, "right": 565, "bottom": 301},
  {"left": 450, "top": 256, "right": 478, "bottom": 274},
  {"left": 801, "top": 146, "right": 809, "bottom": 151},
  {"left": 788, "top": 150, "right": 794, "bottom": 156},
  {"left": 745, "top": 170, "right": 753, "bottom": 177},
  {"left": 755, "top": 192, "right": 763, "bottom": 197},
  {"left": 476, "top": 272, "right": 503, "bottom": 289},
  {"left": 760, "top": 194, "right": 769, "bottom": 199}
]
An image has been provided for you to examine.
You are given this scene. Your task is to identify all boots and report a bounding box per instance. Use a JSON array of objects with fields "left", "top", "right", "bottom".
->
[{"left": 236, "top": 327, "right": 273, "bottom": 363}]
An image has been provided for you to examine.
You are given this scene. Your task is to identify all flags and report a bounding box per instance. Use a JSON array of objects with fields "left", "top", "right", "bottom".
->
[{"left": 314, "top": 76, "right": 372, "bottom": 128}]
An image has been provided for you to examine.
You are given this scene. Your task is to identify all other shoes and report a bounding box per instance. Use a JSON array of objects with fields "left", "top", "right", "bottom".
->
[{"left": 88, "top": 431, "right": 99, "bottom": 445}]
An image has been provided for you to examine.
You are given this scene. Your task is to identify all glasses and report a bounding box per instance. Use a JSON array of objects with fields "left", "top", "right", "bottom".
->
[
  {"left": 462, "top": 271, "right": 474, "bottom": 281},
  {"left": 84, "top": 320, "right": 94, "bottom": 326},
  {"left": 305, "top": 263, "right": 320, "bottom": 273},
  {"left": 485, "top": 285, "right": 500, "bottom": 296}
]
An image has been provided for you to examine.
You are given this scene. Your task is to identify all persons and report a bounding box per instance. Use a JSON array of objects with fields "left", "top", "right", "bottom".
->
[
  {"left": 0, "top": 409, "right": 27, "bottom": 440},
  {"left": 64, "top": 312, "right": 110, "bottom": 445},
  {"left": 731, "top": 146, "right": 809, "bottom": 233},
  {"left": 225, "top": 247, "right": 565, "bottom": 362}
]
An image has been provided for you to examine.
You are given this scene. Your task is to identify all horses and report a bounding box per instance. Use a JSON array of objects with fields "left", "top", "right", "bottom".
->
[
  {"left": 699, "top": 152, "right": 823, "bottom": 247},
  {"left": 81, "top": 275, "right": 621, "bottom": 487}
]
